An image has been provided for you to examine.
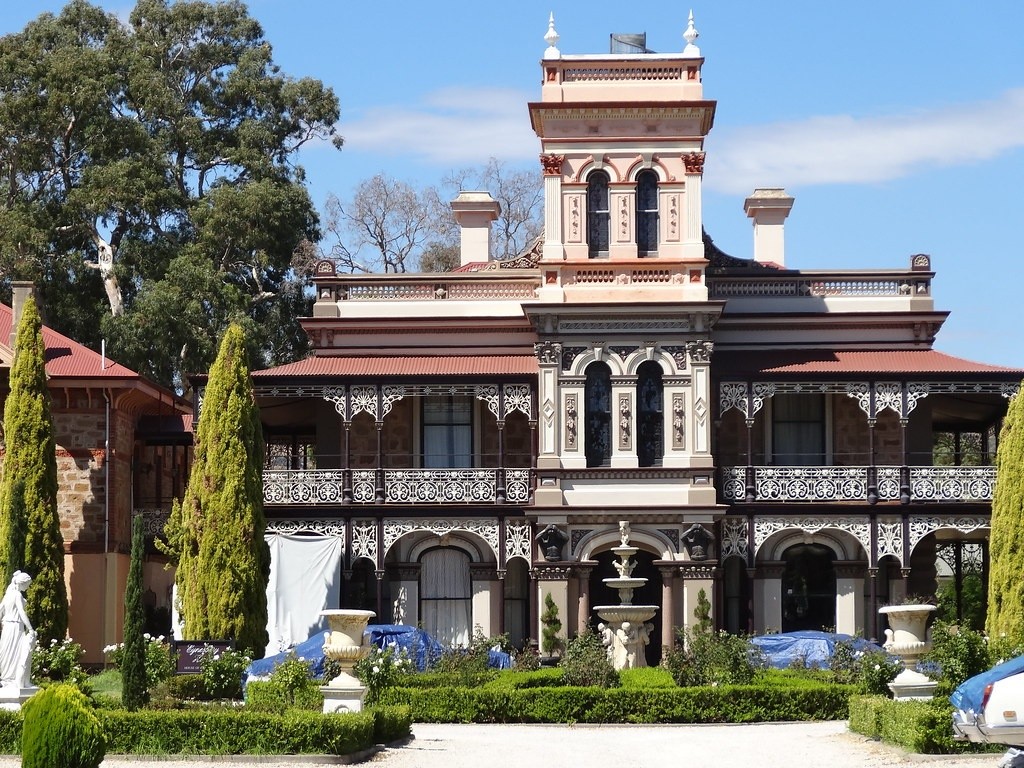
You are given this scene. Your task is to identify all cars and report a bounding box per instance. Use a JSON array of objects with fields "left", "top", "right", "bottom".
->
[
  {"left": 950, "top": 653, "right": 1024, "bottom": 751},
  {"left": 241, "top": 625, "right": 509, "bottom": 706},
  {"left": 747, "top": 631, "right": 941, "bottom": 676}
]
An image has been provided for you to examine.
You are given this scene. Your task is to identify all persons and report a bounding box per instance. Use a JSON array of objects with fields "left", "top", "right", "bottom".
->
[
  {"left": 535, "top": 525, "right": 569, "bottom": 561},
  {"left": 681, "top": 523, "right": 715, "bottom": 560},
  {"left": 638, "top": 623, "right": 654, "bottom": 666},
  {"left": 0, "top": 570, "right": 35, "bottom": 686},
  {"left": 615, "top": 622, "right": 634, "bottom": 670},
  {"left": 598, "top": 623, "right": 614, "bottom": 666}
]
